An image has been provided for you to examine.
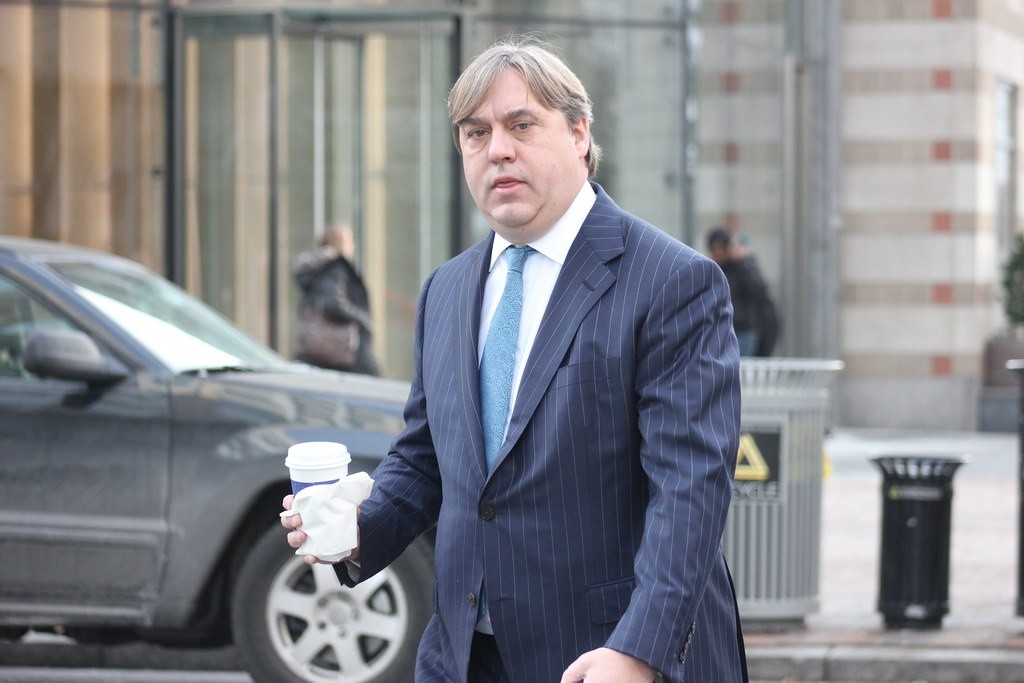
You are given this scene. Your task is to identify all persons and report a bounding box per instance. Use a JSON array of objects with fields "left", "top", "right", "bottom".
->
[
  {"left": 280, "top": 41, "right": 748, "bottom": 682},
  {"left": 288, "top": 220, "right": 382, "bottom": 375},
  {"left": 705, "top": 226, "right": 787, "bottom": 359}
]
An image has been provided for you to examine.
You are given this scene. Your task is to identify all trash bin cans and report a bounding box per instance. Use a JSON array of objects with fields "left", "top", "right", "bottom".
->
[
  {"left": 872, "top": 453, "right": 963, "bottom": 631},
  {"left": 717, "top": 356, "right": 846, "bottom": 633}
]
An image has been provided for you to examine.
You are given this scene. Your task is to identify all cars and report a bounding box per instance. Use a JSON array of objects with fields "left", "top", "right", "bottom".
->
[{"left": 0, "top": 235, "right": 437, "bottom": 683}]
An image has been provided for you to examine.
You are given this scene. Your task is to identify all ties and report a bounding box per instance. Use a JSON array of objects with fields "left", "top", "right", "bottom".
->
[{"left": 477, "top": 246, "right": 533, "bottom": 479}]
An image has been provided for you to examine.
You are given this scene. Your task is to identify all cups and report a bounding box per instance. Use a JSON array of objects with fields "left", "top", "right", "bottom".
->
[{"left": 284, "top": 442, "right": 351, "bottom": 501}]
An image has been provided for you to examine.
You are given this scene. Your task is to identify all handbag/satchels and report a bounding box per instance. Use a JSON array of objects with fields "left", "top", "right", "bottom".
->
[{"left": 294, "top": 308, "right": 362, "bottom": 372}]
